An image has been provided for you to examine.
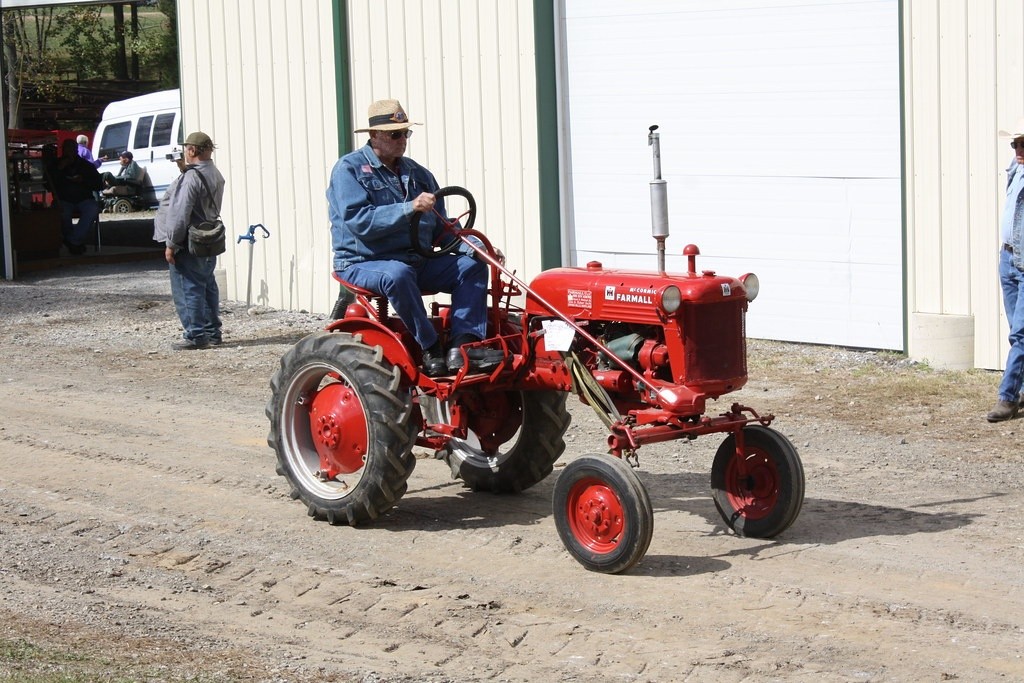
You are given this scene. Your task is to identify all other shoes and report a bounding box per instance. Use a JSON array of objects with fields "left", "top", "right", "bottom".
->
[
  {"left": 171, "top": 339, "right": 209, "bottom": 350},
  {"left": 63, "top": 237, "right": 80, "bottom": 256},
  {"left": 77, "top": 242, "right": 87, "bottom": 253},
  {"left": 205, "top": 335, "right": 222, "bottom": 345}
]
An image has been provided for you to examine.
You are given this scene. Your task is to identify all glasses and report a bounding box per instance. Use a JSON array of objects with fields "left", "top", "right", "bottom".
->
[
  {"left": 380, "top": 129, "right": 413, "bottom": 140},
  {"left": 1010, "top": 142, "right": 1024, "bottom": 150}
]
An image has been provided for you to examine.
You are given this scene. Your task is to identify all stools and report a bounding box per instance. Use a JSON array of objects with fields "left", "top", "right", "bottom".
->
[{"left": 334, "top": 271, "right": 439, "bottom": 297}]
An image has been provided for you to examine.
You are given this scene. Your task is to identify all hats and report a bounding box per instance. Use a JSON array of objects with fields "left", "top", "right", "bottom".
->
[
  {"left": 119, "top": 151, "right": 133, "bottom": 158},
  {"left": 353, "top": 99, "right": 424, "bottom": 133},
  {"left": 999, "top": 121, "right": 1024, "bottom": 140},
  {"left": 177, "top": 131, "right": 213, "bottom": 147}
]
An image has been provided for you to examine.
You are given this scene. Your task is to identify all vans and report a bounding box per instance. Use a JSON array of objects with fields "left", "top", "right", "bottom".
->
[
  {"left": 23, "top": 129, "right": 94, "bottom": 206},
  {"left": 91, "top": 88, "right": 185, "bottom": 207}
]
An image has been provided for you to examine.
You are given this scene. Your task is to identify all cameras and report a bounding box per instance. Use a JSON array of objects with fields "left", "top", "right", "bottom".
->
[{"left": 165, "top": 151, "right": 184, "bottom": 162}]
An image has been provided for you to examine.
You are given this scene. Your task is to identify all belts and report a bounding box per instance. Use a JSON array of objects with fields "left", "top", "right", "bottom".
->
[{"left": 1005, "top": 242, "right": 1013, "bottom": 254}]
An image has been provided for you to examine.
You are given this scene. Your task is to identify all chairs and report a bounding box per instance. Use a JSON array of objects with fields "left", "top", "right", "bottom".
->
[
  {"left": 72, "top": 191, "right": 101, "bottom": 254},
  {"left": 102, "top": 166, "right": 144, "bottom": 214}
]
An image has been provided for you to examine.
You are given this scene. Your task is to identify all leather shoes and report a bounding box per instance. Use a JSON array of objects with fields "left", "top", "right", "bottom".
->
[
  {"left": 1015, "top": 391, "right": 1024, "bottom": 416},
  {"left": 987, "top": 393, "right": 1020, "bottom": 423},
  {"left": 420, "top": 338, "right": 450, "bottom": 377},
  {"left": 445, "top": 344, "right": 514, "bottom": 373}
]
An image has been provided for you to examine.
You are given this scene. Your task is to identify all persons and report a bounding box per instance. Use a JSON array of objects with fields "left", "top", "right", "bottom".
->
[
  {"left": 77, "top": 134, "right": 108, "bottom": 169},
  {"left": 101, "top": 151, "right": 141, "bottom": 193},
  {"left": 325, "top": 100, "right": 512, "bottom": 370},
  {"left": 152, "top": 131, "right": 225, "bottom": 351},
  {"left": 42, "top": 136, "right": 117, "bottom": 254},
  {"left": 987, "top": 133, "right": 1024, "bottom": 422}
]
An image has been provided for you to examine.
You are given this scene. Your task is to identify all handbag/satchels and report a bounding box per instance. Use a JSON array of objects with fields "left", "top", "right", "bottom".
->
[{"left": 187, "top": 217, "right": 226, "bottom": 257}]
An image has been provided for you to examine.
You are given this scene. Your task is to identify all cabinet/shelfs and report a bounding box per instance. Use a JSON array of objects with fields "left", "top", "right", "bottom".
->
[{"left": 8, "top": 157, "right": 64, "bottom": 261}]
